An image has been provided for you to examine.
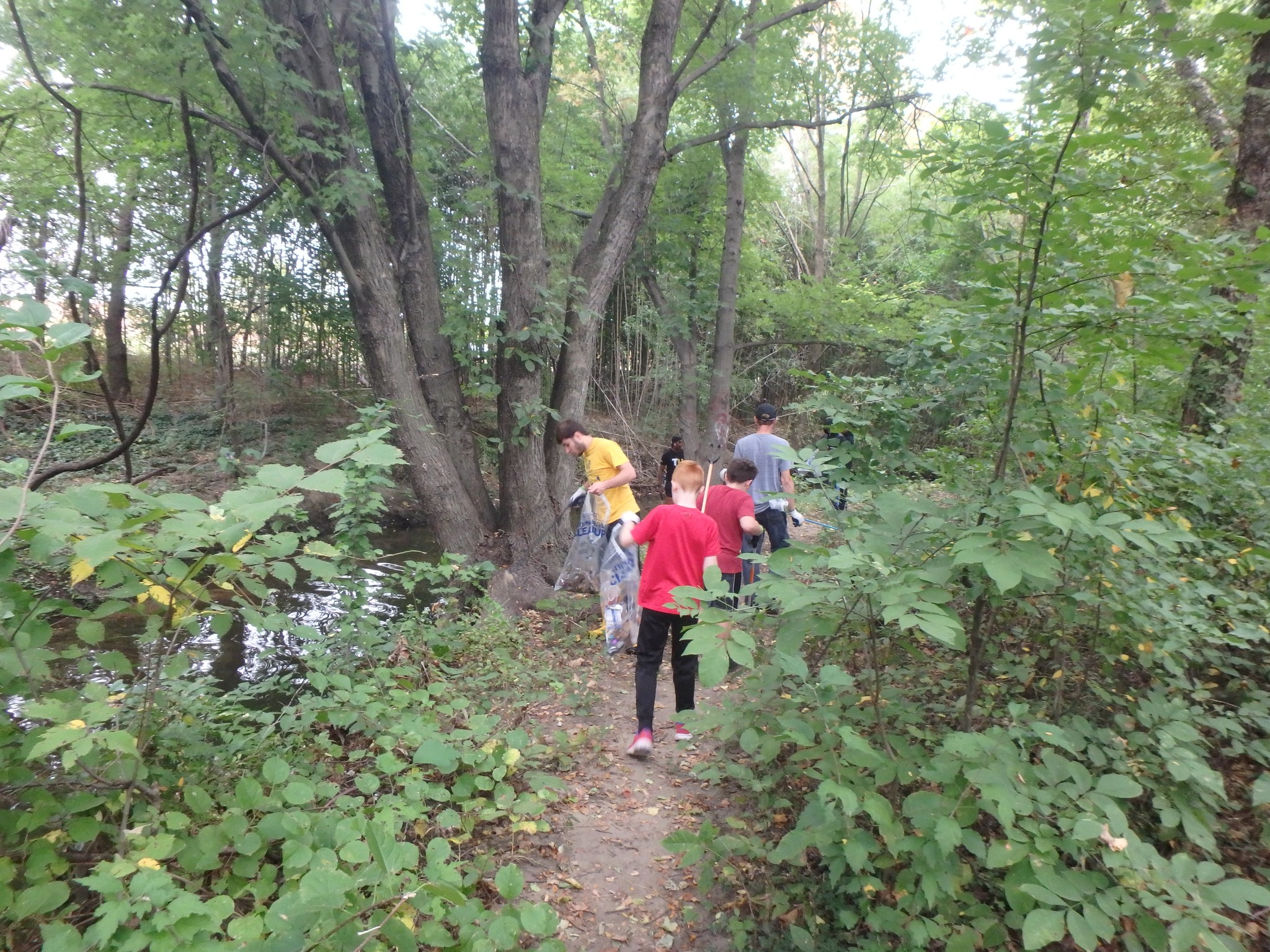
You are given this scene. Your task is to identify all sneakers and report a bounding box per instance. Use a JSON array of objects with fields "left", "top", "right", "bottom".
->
[
  {"left": 694, "top": 651, "right": 738, "bottom": 680},
  {"left": 627, "top": 728, "right": 653, "bottom": 757},
  {"left": 674, "top": 724, "right": 693, "bottom": 741},
  {"left": 759, "top": 606, "right": 778, "bottom": 618}
]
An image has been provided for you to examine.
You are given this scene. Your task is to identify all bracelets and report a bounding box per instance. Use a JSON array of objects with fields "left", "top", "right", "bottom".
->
[{"left": 581, "top": 486, "right": 588, "bottom": 492}]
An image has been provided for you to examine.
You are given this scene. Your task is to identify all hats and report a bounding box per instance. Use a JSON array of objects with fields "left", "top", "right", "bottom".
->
[{"left": 756, "top": 403, "right": 776, "bottom": 422}]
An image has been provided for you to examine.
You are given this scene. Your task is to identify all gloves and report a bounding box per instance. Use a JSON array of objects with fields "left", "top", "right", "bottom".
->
[
  {"left": 719, "top": 468, "right": 727, "bottom": 482},
  {"left": 789, "top": 508, "right": 804, "bottom": 527},
  {"left": 570, "top": 486, "right": 587, "bottom": 509}
]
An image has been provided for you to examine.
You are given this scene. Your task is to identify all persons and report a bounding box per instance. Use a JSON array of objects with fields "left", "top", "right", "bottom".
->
[
  {"left": 815, "top": 418, "right": 854, "bottom": 513},
  {"left": 618, "top": 460, "right": 721, "bottom": 755},
  {"left": 695, "top": 458, "right": 764, "bottom": 673},
  {"left": 657, "top": 436, "right": 685, "bottom": 505},
  {"left": 556, "top": 420, "right": 641, "bottom": 636},
  {"left": 732, "top": 403, "right": 805, "bottom": 617}
]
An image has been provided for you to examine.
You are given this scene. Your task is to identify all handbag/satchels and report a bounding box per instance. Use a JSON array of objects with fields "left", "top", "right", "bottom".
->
[
  {"left": 554, "top": 490, "right": 611, "bottom": 595},
  {"left": 599, "top": 509, "right": 642, "bottom": 656}
]
